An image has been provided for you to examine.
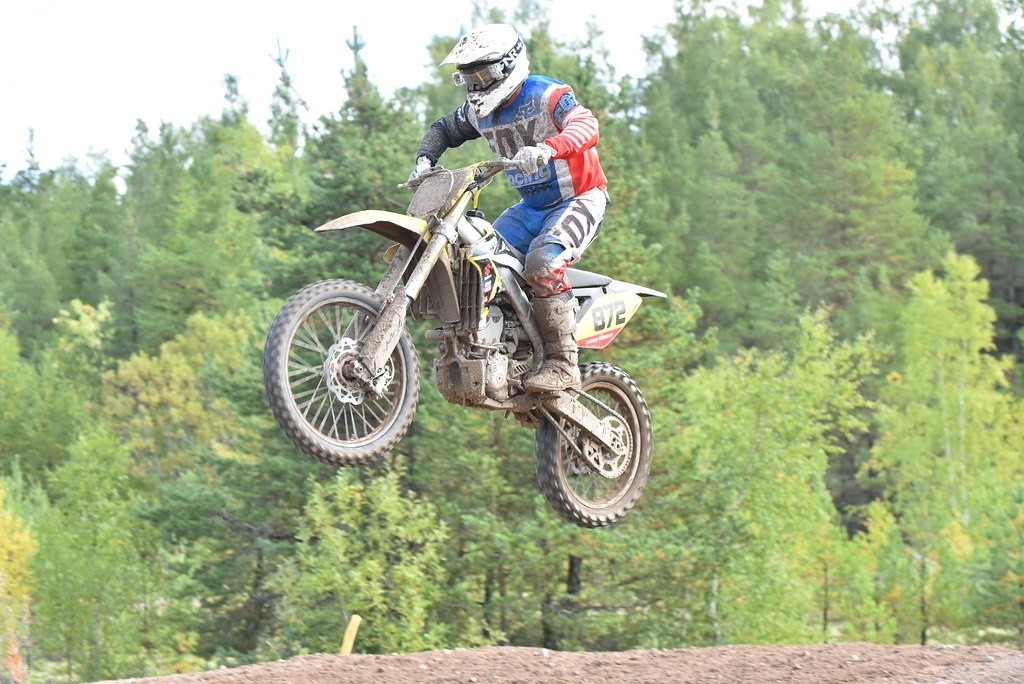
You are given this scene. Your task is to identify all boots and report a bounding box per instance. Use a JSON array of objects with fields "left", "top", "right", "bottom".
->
[{"left": 525, "top": 290, "right": 581, "bottom": 391}]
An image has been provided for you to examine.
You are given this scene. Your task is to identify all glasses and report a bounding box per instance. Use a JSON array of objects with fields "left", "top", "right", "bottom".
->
[{"left": 464, "top": 37, "right": 524, "bottom": 90}]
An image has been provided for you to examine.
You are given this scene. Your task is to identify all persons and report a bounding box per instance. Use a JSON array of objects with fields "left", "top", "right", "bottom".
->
[{"left": 408, "top": 24, "right": 610, "bottom": 392}]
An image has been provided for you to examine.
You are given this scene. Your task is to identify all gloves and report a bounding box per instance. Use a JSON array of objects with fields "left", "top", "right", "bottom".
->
[
  {"left": 408, "top": 156, "right": 432, "bottom": 188},
  {"left": 513, "top": 144, "right": 556, "bottom": 176}
]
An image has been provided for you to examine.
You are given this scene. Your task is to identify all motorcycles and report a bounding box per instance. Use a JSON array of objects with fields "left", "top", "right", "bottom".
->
[{"left": 263, "top": 153, "right": 669, "bottom": 529}]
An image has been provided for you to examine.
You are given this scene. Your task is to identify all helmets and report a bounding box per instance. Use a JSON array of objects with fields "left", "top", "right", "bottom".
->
[{"left": 438, "top": 24, "right": 528, "bottom": 118}]
